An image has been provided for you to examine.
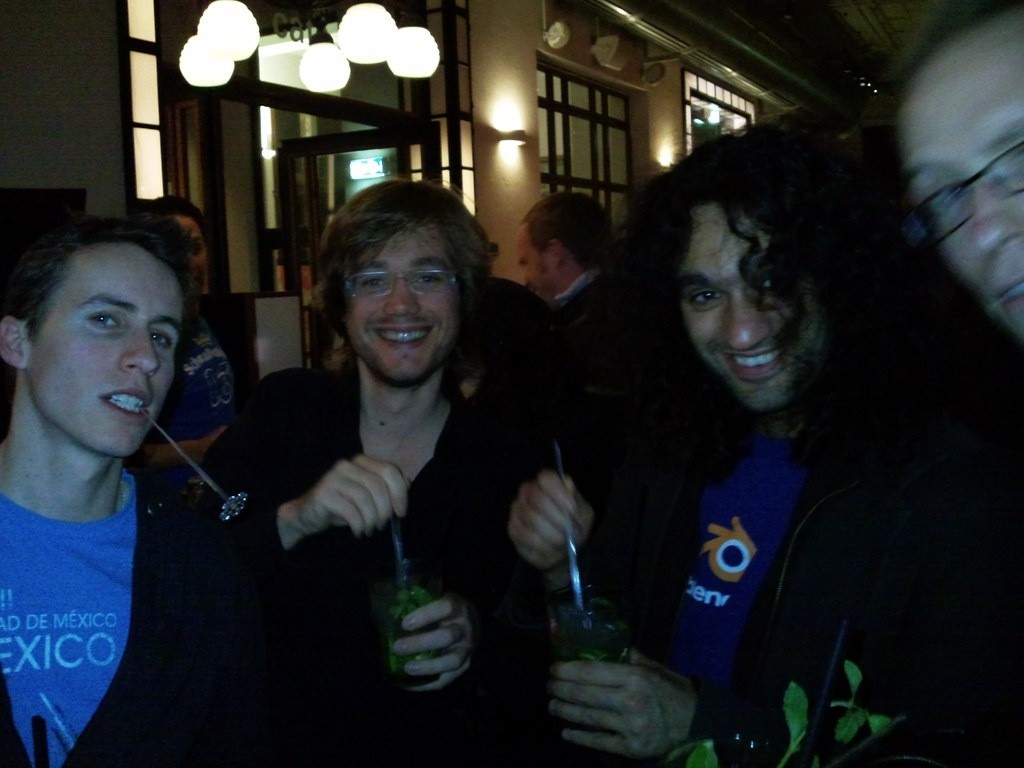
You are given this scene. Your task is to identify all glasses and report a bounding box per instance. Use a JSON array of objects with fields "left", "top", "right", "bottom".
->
[
  {"left": 898, "top": 142, "right": 1024, "bottom": 251},
  {"left": 342, "top": 265, "right": 458, "bottom": 300}
]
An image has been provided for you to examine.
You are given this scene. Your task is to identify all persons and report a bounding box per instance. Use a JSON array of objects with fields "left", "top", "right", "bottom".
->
[
  {"left": 898, "top": 1, "right": 1024, "bottom": 345},
  {"left": 517, "top": 191, "right": 620, "bottom": 320},
  {"left": 198, "top": 170, "right": 541, "bottom": 768},
  {"left": 505, "top": 127, "right": 1024, "bottom": 768},
  {"left": 123, "top": 194, "right": 234, "bottom": 504},
  {"left": 0, "top": 216, "right": 270, "bottom": 768}
]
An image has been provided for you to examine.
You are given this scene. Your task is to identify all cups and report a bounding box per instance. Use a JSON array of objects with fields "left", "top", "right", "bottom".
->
[
  {"left": 544, "top": 589, "right": 636, "bottom": 666},
  {"left": 367, "top": 563, "right": 450, "bottom": 674}
]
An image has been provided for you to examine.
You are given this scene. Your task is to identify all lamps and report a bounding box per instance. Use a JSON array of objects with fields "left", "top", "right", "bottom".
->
[{"left": 639, "top": 62, "right": 667, "bottom": 87}]
